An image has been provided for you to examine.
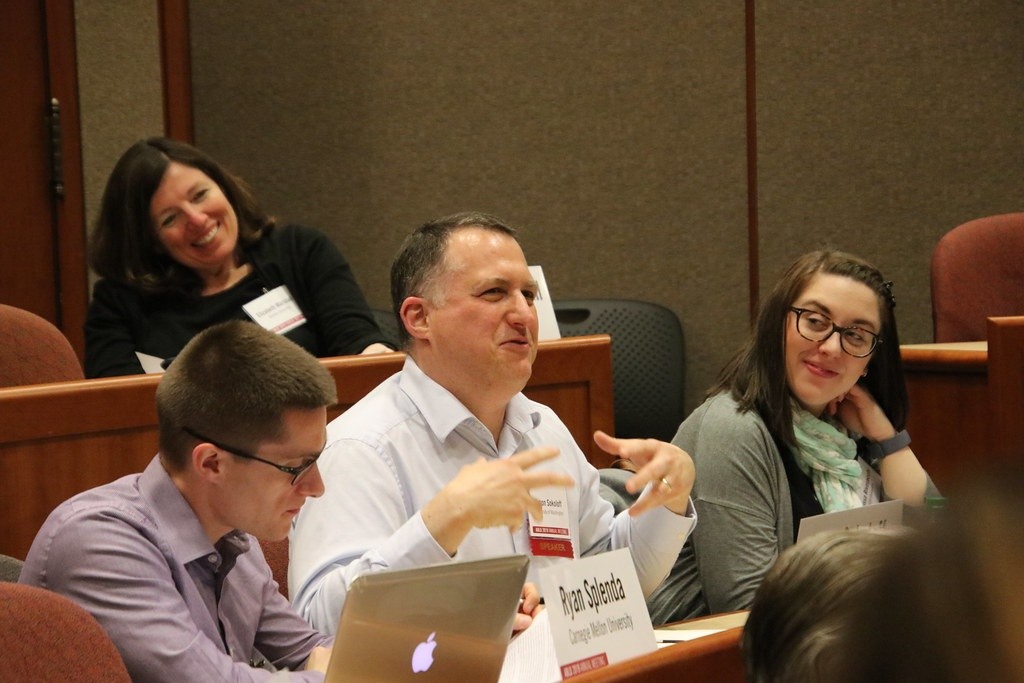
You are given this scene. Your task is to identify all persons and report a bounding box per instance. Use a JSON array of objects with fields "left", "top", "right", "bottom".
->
[
  {"left": 742, "top": 529, "right": 908, "bottom": 683},
  {"left": 17, "top": 322, "right": 544, "bottom": 683},
  {"left": 83, "top": 138, "right": 398, "bottom": 380},
  {"left": 288, "top": 212, "right": 698, "bottom": 658},
  {"left": 646, "top": 250, "right": 946, "bottom": 630}
]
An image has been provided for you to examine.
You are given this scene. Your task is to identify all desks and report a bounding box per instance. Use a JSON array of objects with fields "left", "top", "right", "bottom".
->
[
  {"left": 894, "top": 316, "right": 1024, "bottom": 501},
  {"left": 0, "top": 334, "right": 619, "bottom": 560},
  {"left": 553, "top": 607, "right": 755, "bottom": 683}
]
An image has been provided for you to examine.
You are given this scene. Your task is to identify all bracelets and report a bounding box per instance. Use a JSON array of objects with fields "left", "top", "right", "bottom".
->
[{"left": 862, "top": 428, "right": 913, "bottom": 465}]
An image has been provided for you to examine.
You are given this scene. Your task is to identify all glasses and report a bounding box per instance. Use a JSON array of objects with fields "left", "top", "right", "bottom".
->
[
  {"left": 788, "top": 303, "right": 884, "bottom": 357},
  {"left": 181, "top": 424, "right": 327, "bottom": 485}
]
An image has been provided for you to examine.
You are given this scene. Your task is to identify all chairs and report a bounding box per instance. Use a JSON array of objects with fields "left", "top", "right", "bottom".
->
[
  {"left": 550, "top": 298, "right": 688, "bottom": 443},
  {"left": 0, "top": 550, "right": 136, "bottom": 683},
  {"left": 0, "top": 304, "right": 89, "bottom": 389},
  {"left": 930, "top": 211, "right": 1024, "bottom": 343}
]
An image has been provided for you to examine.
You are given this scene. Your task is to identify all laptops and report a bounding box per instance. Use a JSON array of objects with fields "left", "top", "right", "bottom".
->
[{"left": 321, "top": 555, "right": 532, "bottom": 683}]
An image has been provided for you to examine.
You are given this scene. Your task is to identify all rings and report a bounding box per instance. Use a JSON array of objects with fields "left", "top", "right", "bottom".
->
[{"left": 662, "top": 477, "right": 672, "bottom": 492}]
